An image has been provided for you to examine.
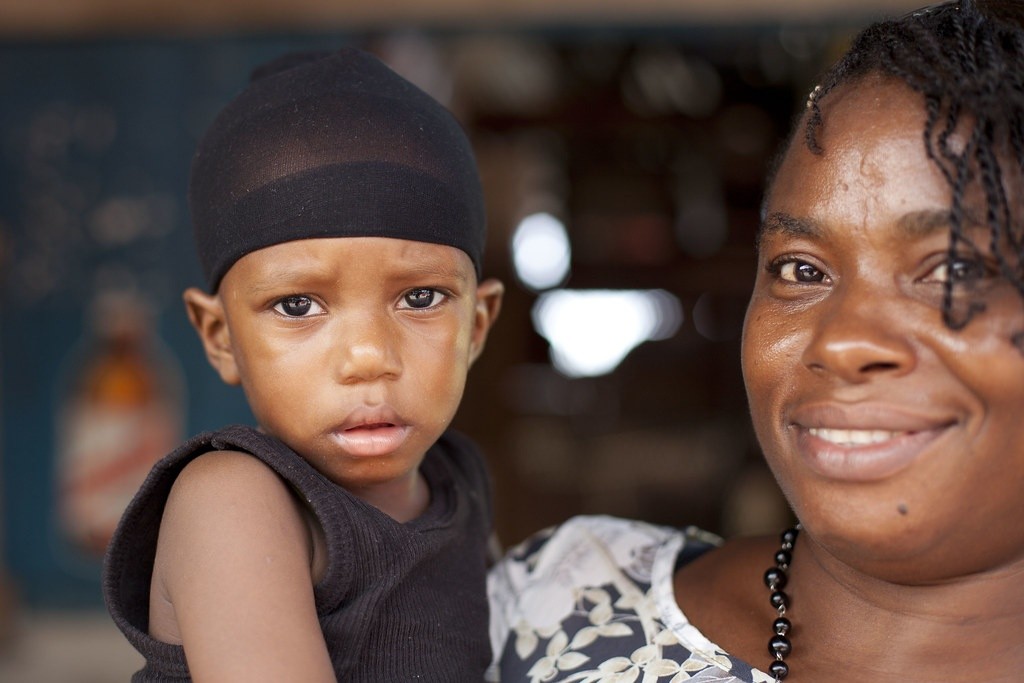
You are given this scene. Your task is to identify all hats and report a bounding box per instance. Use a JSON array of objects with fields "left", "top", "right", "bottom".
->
[{"left": 191, "top": 47, "right": 483, "bottom": 294}]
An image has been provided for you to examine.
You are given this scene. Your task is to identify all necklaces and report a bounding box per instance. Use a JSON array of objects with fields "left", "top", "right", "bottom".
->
[{"left": 764, "top": 521, "right": 802, "bottom": 681}]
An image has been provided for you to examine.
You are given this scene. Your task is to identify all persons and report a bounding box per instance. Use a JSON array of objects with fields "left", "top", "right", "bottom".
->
[
  {"left": 485, "top": 0, "right": 1024, "bottom": 683},
  {"left": 100, "top": 52, "right": 507, "bottom": 683}
]
